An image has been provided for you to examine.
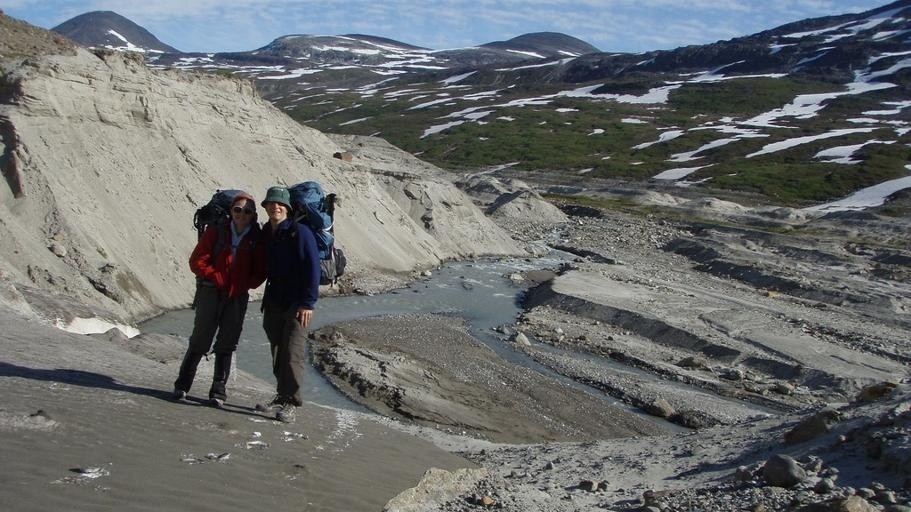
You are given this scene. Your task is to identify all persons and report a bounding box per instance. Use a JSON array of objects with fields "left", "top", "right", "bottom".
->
[
  {"left": 254, "top": 186, "right": 321, "bottom": 423},
  {"left": 173, "top": 192, "right": 268, "bottom": 407}
]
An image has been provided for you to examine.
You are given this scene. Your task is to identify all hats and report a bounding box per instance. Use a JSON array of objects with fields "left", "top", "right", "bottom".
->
[{"left": 261, "top": 187, "right": 292, "bottom": 211}]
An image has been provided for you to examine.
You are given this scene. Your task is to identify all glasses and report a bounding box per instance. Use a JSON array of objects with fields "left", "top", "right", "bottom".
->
[{"left": 234, "top": 206, "right": 252, "bottom": 216}]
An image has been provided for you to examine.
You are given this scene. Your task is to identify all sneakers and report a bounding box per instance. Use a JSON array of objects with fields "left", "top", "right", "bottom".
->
[
  {"left": 209, "top": 398, "right": 223, "bottom": 407},
  {"left": 256, "top": 394, "right": 296, "bottom": 422},
  {"left": 174, "top": 388, "right": 187, "bottom": 399}
]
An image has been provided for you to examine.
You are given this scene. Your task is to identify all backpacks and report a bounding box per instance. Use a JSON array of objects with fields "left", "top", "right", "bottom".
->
[
  {"left": 289, "top": 181, "right": 347, "bottom": 286},
  {"left": 195, "top": 189, "right": 255, "bottom": 255}
]
